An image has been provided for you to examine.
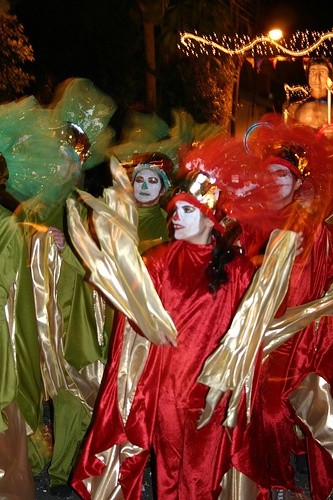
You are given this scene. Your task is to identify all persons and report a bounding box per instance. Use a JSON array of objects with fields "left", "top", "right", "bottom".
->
[
  {"left": 76, "top": 149, "right": 177, "bottom": 259},
  {"left": 0, "top": 152, "right": 21, "bottom": 436},
  {"left": 0, "top": 221, "right": 98, "bottom": 499},
  {"left": 219, "top": 128, "right": 332, "bottom": 499},
  {"left": 70, "top": 170, "right": 303, "bottom": 500},
  {"left": 11, "top": 121, "right": 97, "bottom": 494}
]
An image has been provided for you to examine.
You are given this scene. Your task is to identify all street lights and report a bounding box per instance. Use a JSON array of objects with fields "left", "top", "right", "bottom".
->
[{"left": 251, "top": 29, "right": 282, "bottom": 117}]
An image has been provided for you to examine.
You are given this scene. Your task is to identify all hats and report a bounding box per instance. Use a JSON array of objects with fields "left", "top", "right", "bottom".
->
[
  {"left": 0, "top": 94, "right": 82, "bottom": 220},
  {"left": 47, "top": 77, "right": 117, "bottom": 173},
  {"left": 164, "top": 132, "right": 283, "bottom": 259},
  {"left": 247, "top": 112, "right": 333, "bottom": 193},
  {"left": 113, "top": 109, "right": 223, "bottom": 193}
]
